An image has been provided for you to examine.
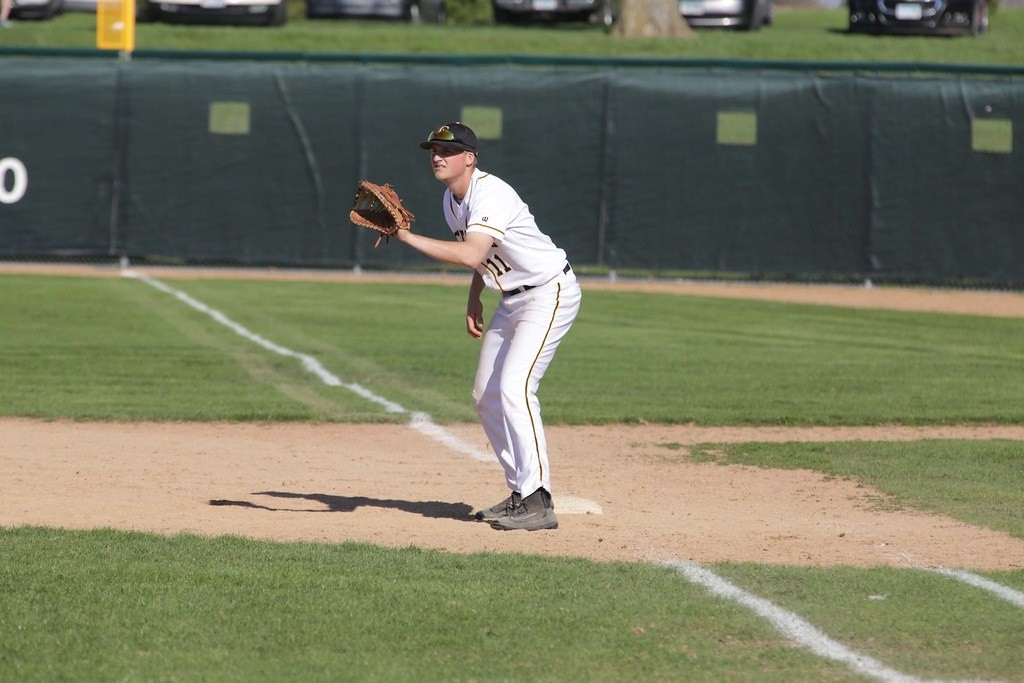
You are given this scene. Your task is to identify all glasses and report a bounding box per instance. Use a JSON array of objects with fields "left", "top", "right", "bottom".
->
[{"left": 428, "top": 130, "right": 476, "bottom": 150}]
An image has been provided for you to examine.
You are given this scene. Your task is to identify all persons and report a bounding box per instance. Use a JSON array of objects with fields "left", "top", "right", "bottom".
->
[{"left": 349, "top": 122, "right": 582, "bottom": 529}]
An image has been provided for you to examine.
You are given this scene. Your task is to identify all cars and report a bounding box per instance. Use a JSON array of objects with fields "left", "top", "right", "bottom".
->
[
  {"left": 492, "top": 0, "right": 621, "bottom": 32},
  {"left": 677, "top": 0, "right": 775, "bottom": 30},
  {"left": 846, "top": 0, "right": 992, "bottom": 36},
  {"left": 9, "top": 0, "right": 65, "bottom": 20},
  {"left": 136, "top": 0, "right": 288, "bottom": 26},
  {"left": 306, "top": 0, "right": 451, "bottom": 24}
]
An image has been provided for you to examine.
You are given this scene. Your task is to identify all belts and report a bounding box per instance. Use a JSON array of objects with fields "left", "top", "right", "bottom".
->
[{"left": 508, "top": 262, "right": 570, "bottom": 295}]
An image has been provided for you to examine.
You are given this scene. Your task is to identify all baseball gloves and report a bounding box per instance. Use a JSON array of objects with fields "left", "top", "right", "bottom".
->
[{"left": 349, "top": 180, "right": 411, "bottom": 237}]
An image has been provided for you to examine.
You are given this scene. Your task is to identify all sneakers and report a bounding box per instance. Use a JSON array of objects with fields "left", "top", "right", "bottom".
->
[
  {"left": 492, "top": 489, "right": 558, "bottom": 531},
  {"left": 476, "top": 491, "right": 521, "bottom": 518}
]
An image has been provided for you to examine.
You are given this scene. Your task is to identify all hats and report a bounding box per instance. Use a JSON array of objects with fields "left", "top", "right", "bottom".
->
[{"left": 421, "top": 122, "right": 479, "bottom": 158}]
